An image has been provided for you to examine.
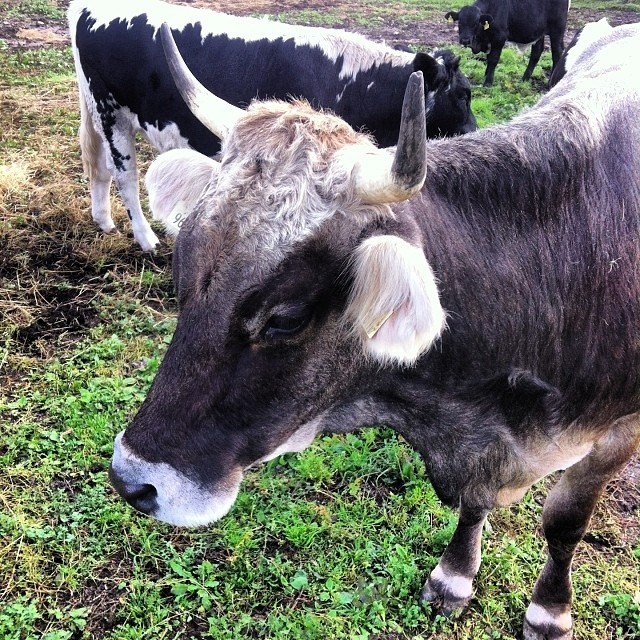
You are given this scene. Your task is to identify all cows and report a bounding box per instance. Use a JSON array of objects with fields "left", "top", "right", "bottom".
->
[
  {"left": 65, "top": 0, "right": 480, "bottom": 254},
  {"left": 107, "top": 18, "right": 640, "bottom": 640},
  {"left": 445, "top": 0, "right": 570, "bottom": 88}
]
